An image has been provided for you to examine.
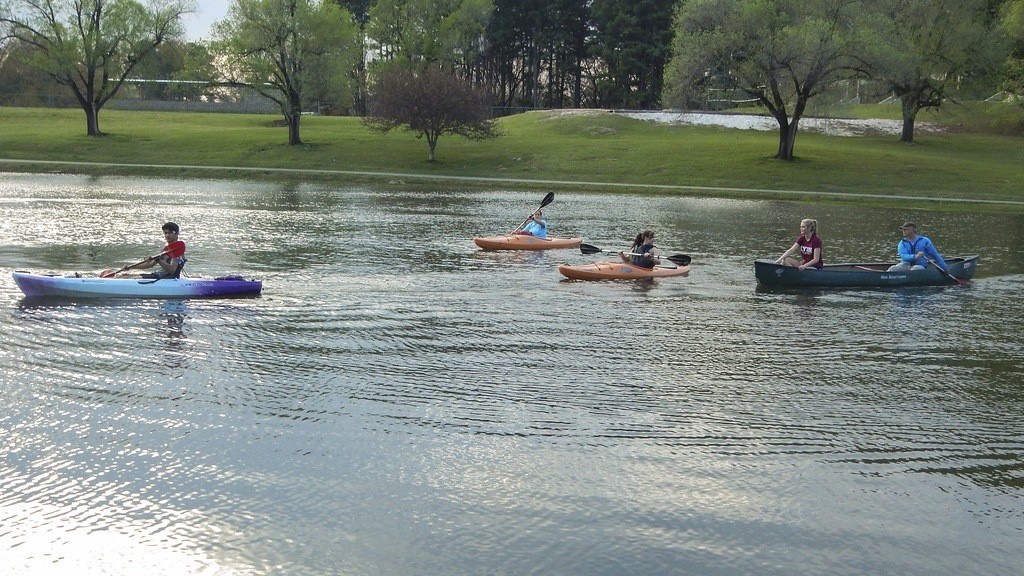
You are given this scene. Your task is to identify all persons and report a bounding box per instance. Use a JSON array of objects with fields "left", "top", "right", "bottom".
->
[
  {"left": 773, "top": 218, "right": 823, "bottom": 272},
  {"left": 886, "top": 222, "right": 950, "bottom": 275},
  {"left": 75, "top": 222, "right": 185, "bottom": 279},
  {"left": 617, "top": 230, "right": 661, "bottom": 268},
  {"left": 511, "top": 209, "right": 547, "bottom": 238}
]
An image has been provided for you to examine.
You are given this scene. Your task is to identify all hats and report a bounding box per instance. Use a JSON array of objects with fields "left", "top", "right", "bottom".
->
[{"left": 899, "top": 222, "right": 916, "bottom": 229}]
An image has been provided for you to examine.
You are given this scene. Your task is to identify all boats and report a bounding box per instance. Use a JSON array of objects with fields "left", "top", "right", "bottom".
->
[
  {"left": 753, "top": 254, "right": 980, "bottom": 284},
  {"left": 473, "top": 236, "right": 582, "bottom": 249},
  {"left": 559, "top": 254, "right": 691, "bottom": 279},
  {"left": 11, "top": 270, "right": 264, "bottom": 300}
]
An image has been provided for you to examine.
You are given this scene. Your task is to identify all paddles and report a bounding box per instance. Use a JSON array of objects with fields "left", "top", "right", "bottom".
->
[
  {"left": 580, "top": 242, "right": 692, "bottom": 267},
  {"left": 100, "top": 249, "right": 173, "bottom": 278},
  {"left": 512, "top": 192, "right": 555, "bottom": 234},
  {"left": 922, "top": 256, "right": 970, "bottom": 285}
]
